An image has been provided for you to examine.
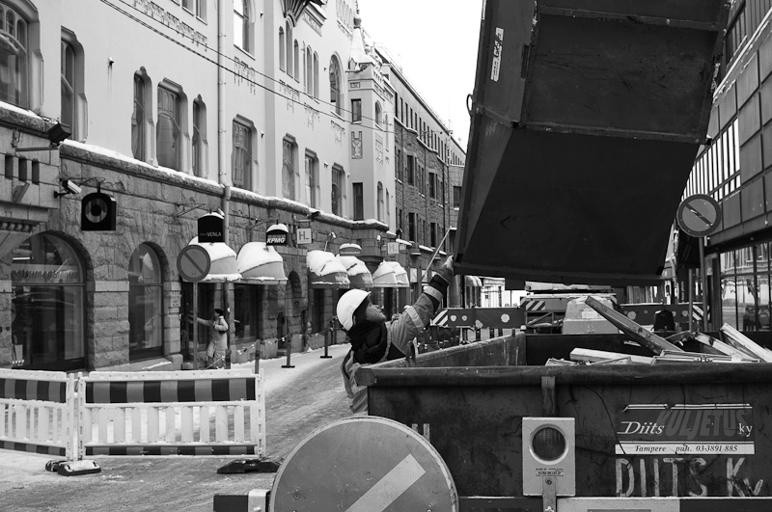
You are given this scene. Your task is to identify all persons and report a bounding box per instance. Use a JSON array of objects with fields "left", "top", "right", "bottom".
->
[
  {"left": 299, "top": 317, "right": 318, "bottom": 352},
  {"left": 181, "top": 301, "right": 193, "bottom": 361},
  {"left": 197, "top": 308, "right": 230, "bottom": 367},
  {"left": 743, "top": 307, "right": 762, "bottom": 331},
  {"left": 336, "top": 256, "right": 453, "bottom": 415}
]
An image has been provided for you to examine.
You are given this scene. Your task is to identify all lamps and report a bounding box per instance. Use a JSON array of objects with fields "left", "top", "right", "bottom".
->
[
  {"left": 12, "top": 181, "right": 29, "bottom": 205},
  {"left": 49, "top": 123, "right": 72, "bottom": 149}
]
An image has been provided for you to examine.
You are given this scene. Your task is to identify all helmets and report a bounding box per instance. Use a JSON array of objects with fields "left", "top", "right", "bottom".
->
[{"left": 337, "top": 289, "right": 372, "bottom": 332}]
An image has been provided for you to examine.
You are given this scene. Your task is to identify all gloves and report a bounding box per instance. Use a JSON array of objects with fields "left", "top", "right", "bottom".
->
[{"left": 443, "top": 254, "right": 455, "bottom": 274}]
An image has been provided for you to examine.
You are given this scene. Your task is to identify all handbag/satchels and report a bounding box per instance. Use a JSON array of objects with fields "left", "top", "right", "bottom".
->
[{"left": 218, "top": 317, "right": 230, "bottom": 335}]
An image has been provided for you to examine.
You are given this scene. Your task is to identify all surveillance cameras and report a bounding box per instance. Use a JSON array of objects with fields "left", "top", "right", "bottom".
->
[
  {"left": 217, "top": 208, "right": 225, "bottom": 217},
  {"left": 62, "top": 179, "right": 82, "bottom": 194},
  {"left": 332, "top": 232, "right": 337, "bottom": 239},
  {"left": 376, "top": 234, "right": 380, "bottom": 242}
]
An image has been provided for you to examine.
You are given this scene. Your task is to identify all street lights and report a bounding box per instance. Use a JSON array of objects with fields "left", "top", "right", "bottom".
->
[{"left": 417, "top": 226, "right": 458, "bottom": 298}]
